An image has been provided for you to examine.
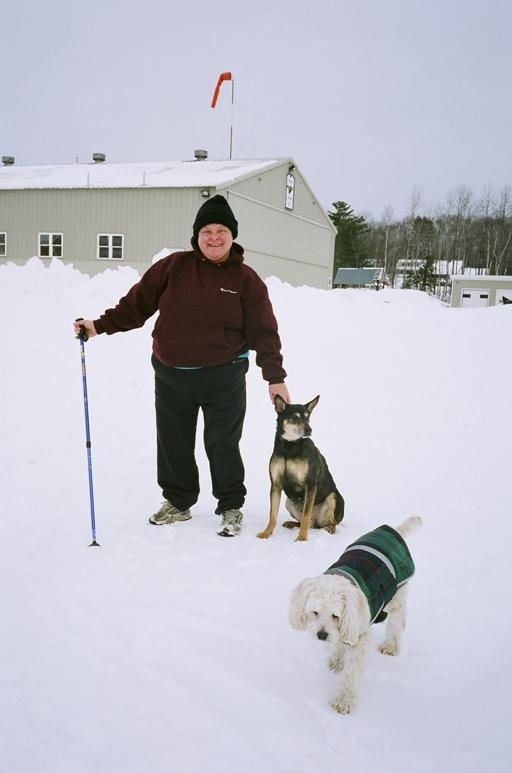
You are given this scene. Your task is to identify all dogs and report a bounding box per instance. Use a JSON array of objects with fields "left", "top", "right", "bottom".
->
[
  {"left": 257, "top": 393, "right": 344, "bottom": 541},
  {"left": 287, "top": 517, "right": 421, "bottom": 714}
]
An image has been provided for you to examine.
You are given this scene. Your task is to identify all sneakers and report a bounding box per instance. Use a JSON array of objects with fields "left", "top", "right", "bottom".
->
[
  {"left": 216, "top": 509, "right": 244, "bottom": 537},
  {"left": 148, "top": 501, "right": 192, "bottom": 526}
]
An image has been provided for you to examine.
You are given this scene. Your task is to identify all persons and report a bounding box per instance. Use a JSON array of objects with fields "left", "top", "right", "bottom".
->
[{"left": 73, "top": 194, "right": 290, "bottom": 537}]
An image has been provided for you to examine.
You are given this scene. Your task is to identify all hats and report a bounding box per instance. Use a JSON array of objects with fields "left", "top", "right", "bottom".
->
[{"left": 193, "top": 195, "right": 238, "bottom": 239}]
